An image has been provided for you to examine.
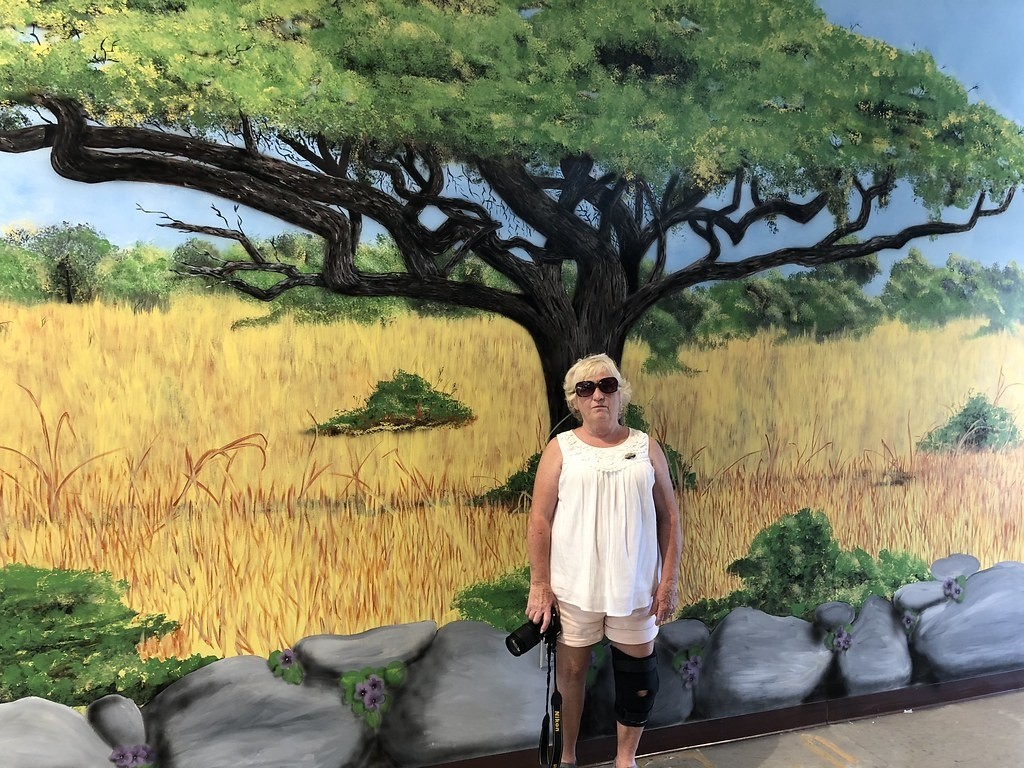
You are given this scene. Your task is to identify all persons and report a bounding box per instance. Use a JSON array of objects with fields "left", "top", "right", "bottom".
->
[{"left": 525, "top": 353, "right": 682, "bottom": 768}]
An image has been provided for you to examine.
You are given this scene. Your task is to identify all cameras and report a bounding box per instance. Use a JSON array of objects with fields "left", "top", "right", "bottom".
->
[{"left": 505, "top": 606, "right": 562, "bottom": 658}]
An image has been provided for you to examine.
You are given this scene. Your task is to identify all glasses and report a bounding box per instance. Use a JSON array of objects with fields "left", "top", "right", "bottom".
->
[{"left": 574, "top": 377, "right": 619, "bottom": 397}]
